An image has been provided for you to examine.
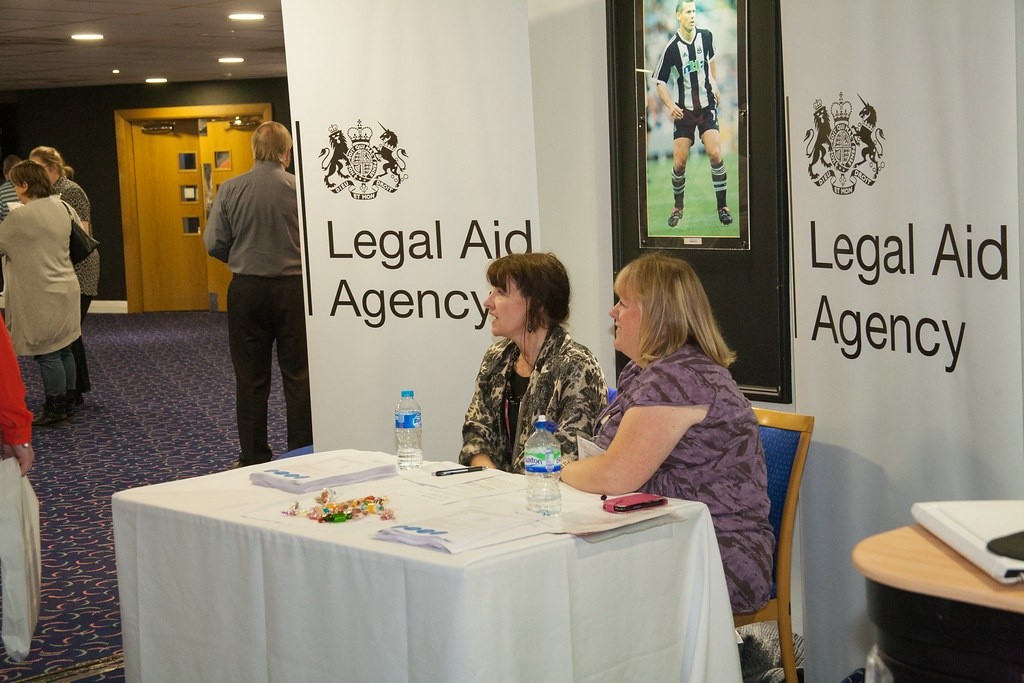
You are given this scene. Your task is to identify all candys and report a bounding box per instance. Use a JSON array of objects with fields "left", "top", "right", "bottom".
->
[{"left": 283, "top": 487, "right": 394, "bottom": 524}]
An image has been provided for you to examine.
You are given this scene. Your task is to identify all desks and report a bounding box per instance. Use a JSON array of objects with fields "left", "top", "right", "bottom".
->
[{"left": 111, "top": 448, "right": 745, "bottom": 683}]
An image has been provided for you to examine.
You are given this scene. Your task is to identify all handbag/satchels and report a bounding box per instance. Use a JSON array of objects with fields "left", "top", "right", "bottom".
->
[
  {"left": 64, "top": 201, "right": 100, "bottom": 266},
  {"left": 0, "top": 456, "right": 42, "bottom": 662}
]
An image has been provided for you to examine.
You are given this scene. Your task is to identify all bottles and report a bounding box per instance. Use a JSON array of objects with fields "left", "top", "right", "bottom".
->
[
  {"left": 524, "top": 415, "right": 562, "bottom": 517},
  {"left": 395, "top": 390, "right": 423, "bottom": 470}
]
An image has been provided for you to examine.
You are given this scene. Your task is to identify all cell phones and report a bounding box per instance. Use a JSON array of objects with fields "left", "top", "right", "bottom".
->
[{"left": 603, "top": 501, "right": 662, "bottom": 511}]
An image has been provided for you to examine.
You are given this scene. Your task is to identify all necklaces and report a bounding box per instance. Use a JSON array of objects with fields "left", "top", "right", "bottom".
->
[{"left": 525, "top": 357, "right": 534, "bottom": 368}]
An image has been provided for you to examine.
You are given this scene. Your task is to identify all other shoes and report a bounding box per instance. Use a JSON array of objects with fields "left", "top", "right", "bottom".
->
[{"left": 76, "top": 391, "right": 84, "bottom": 405}]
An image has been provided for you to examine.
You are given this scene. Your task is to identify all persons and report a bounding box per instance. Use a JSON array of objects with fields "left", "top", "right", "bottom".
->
[
  {"left": 458, "top": 254, "right": 610, "bottom": 475},
  {"left": 0, "top": 145, "right": 100, "bottom": 425},
  {"left": 652, "top": 0, "right": 733, "bottom": 227},
  {"left": 203, "top": 122, "right": 313, "bottom": 466},
  {"left": 0, "top": 314, "right": 35, "bottom": 478},
  {"left": 557, "top": 253, "right": 774, "bottom": 612}
]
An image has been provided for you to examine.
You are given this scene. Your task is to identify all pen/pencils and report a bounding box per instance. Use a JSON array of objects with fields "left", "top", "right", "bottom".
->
[{"left": 435, "top": 466, "right": 489, "bottom": 476}]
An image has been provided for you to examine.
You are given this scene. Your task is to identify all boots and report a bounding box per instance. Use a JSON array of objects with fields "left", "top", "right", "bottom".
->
[
  {"left": 31, "top": 395, "right": 67, "bottom": 429},
  {"left": 64, "top": 386, "right": 79, "bottom": 423}
]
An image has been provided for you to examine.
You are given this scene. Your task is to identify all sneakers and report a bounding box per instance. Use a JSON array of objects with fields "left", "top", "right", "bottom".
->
[
  {"left": 668, "top": 206, "right": 684, "bottom": 227},
  {"left": 712, "top": 205, "right": 733, "bottom": 225}
]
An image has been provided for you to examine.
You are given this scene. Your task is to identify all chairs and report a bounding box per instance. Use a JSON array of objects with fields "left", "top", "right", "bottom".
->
[{"left": 724, "top": 406, "right": 814, "bottom": 683}]
